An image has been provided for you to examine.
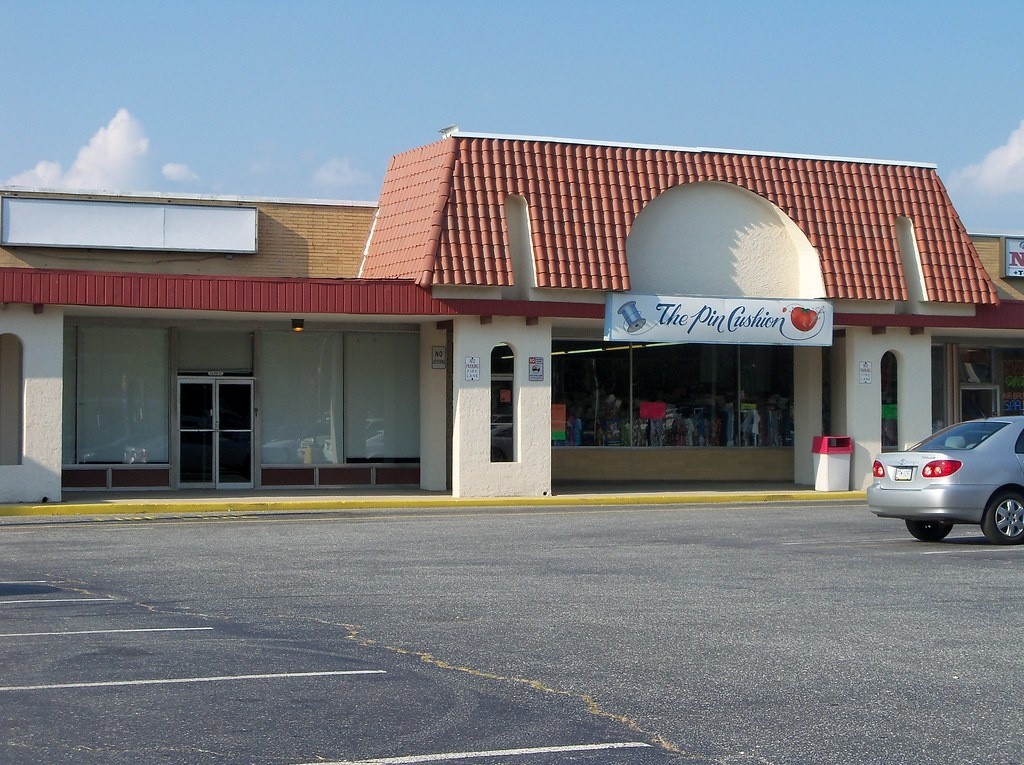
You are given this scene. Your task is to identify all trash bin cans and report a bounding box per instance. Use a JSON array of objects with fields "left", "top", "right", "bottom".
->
[{"left": 811, "top": 434, "right": 854, "bottom": 493}]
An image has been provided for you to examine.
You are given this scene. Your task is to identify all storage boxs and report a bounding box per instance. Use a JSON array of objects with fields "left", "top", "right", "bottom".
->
[
  {"left": 740, "top": 404, "right": 756, "bottom": 410},
  {"left": 500, "top": 390, "right": 511, "bottom": 402}
]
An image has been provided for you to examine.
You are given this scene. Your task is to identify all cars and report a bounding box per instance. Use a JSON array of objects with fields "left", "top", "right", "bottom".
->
[
  {"left": 491, "top": 424, "right": 513, "bottom": 462},
  {"left": 866, "top": 415, "right": 1024, "bottom": 545}
]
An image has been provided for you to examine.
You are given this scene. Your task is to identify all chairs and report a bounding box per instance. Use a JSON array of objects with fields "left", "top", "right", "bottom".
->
[{"left": 945, "top": 436, "right": 965, "bottom": 449}]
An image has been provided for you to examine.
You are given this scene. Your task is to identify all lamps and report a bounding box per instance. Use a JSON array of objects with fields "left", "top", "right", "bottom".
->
[{"left": 292, "top": 318, "right": 305, "bottom": 333}]
